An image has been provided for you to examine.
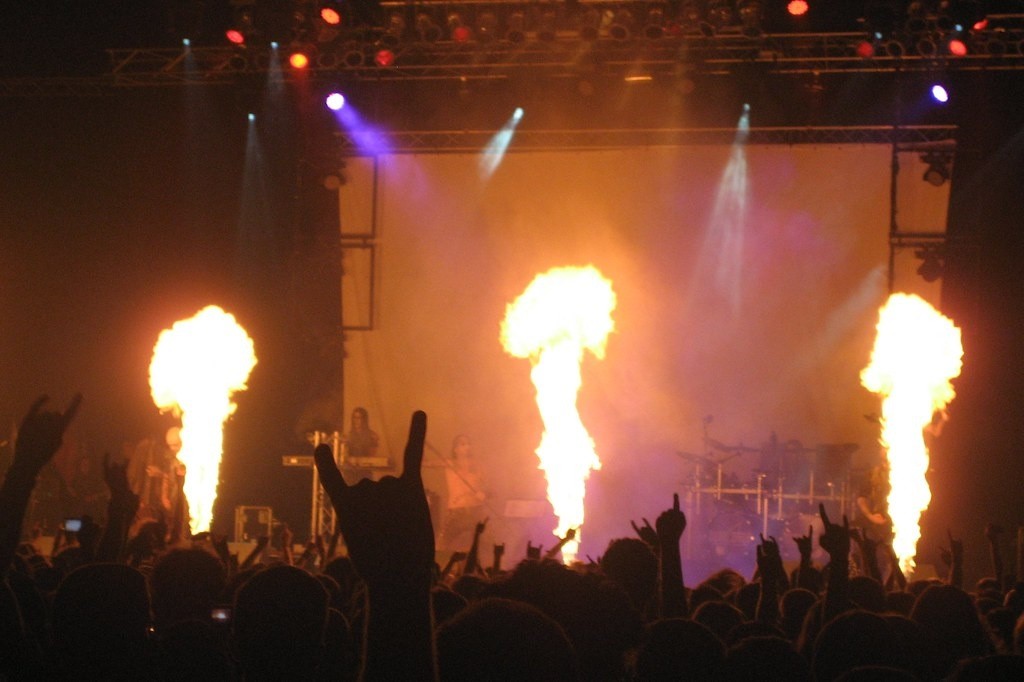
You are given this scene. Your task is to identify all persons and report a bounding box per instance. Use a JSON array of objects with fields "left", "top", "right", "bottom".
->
[
  {"left": 422, "top": 434, "right": 495, "bottom": 547},
  {"left": 342, "top": 407, "right": 380, "bottom": 488},
  {"left": 1, "top": 392, "right": 1024, "bottom": 682}
]
